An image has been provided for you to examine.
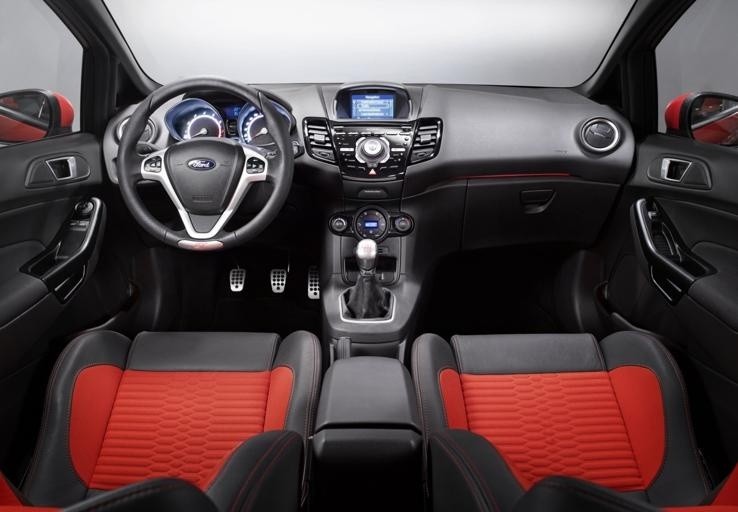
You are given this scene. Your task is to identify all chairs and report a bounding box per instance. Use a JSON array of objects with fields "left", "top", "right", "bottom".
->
[
  {"left": 412, "top": 332, "right": 738, "bottom": 512},
  {"left": 0, "top": 331, "right": 323, "bottom": 512}
]
